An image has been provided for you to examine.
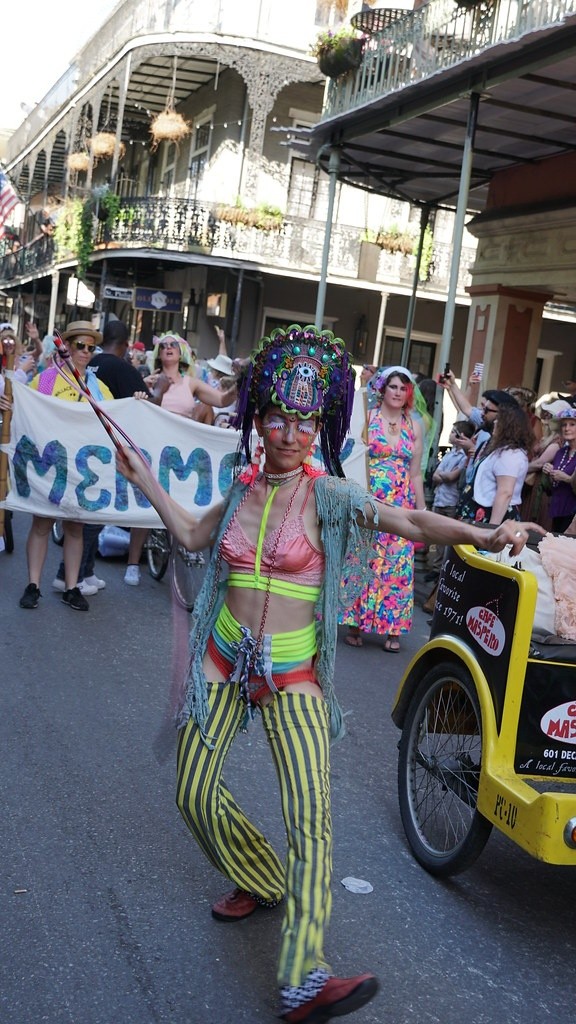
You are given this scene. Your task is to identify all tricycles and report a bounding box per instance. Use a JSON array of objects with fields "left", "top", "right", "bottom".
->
[
  {"left": 51, "top": 518, "right": 213, "bottom": 614},
  {"left": 390, "top": 525, "right": 576, "bottom": 877}
]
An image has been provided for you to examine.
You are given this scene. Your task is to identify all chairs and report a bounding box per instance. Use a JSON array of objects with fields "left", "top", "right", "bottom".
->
[{"left": 427, "top": 520, "right": 576, "bottom": 781}]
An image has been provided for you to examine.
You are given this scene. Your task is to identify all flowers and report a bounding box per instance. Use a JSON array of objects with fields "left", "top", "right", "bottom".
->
[{"left": 306, "top": 30, "right": 355, "bottom": 57}]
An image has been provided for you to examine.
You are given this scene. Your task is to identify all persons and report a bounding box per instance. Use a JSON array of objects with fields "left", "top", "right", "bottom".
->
[
  {"left": 0, "top": 195, "right": 61, "bottom": 283},
  {"left": 0, "top": 321, "right": 248, "bottom": 611},
  {"left": 116, "top": 324, "right": 547, "bottom": 1024},
  {"left": 338, "top": 365, "right": 576, "bottom": 652}
]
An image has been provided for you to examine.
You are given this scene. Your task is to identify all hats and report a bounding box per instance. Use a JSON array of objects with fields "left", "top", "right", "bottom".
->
[
  {"left": 60, "top": 320, "right": 103, "bottom": 345},
  {"left": 541, "top": 399, "right": 571, "bottom": 415},
  {"left": 206, "top": 355, "right": 235, "bottom": 376},
  {"left": 0, "top": 323, "right": 14, "bottom": 332},
  {"left": 132, "top": 342, "right": 146, "bottom": 352},
  {"left": 554, "top": 408, "right": 576, "bottom": 419}
]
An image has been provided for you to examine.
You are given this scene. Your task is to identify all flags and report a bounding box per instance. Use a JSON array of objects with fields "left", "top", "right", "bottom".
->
[{"left": 0, "top": 164, "right": 19, "bottom": 228}]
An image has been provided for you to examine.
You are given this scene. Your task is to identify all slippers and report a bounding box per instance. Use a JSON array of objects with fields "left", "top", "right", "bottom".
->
[
  {"left": 384, "top": 634, "right": 401, "bottom": 653},
  {"left": 344, "top": 631, "right": 362, "bottom": 647}
]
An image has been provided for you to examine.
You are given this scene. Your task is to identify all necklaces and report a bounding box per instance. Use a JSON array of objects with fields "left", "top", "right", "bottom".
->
[
  {"left": 553, "top": 446, "right": 575, "bottom": 488},
  {"left": 263, "top": 463, "right": 303, "bottom": 478},
  {"left": 382, "top": 413, "right": 402, "bottom": 435}
]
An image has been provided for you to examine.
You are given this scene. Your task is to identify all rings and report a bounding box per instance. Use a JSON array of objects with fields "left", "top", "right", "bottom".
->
[{"left": 516, "top": 531, "right": 522, "bottom": 537}]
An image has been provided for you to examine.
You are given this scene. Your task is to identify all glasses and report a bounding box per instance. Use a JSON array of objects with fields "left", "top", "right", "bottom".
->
[
  {"left": 158, "top": 341, "right": 180, "bottom": 351},
  {"left": 0, "top": 327, "right": 14, "bottom": 333},
  {"left": 71, "top": 340, "right": 96, "bottom": 352},
  {"left": 483, "top": 407, "right": 498, "bottom": 415},
  {"left": 2, "top": 340, "right": 14, "bottom": 344},
  {"left": 363, "top": 364, "right": 375, "bottom": 374}
]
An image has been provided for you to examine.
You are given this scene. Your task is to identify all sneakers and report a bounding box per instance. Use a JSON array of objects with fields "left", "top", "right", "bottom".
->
[
  {"left": 51, "top": 578, "right": 98, "bottom": 595},
  {"left": 83, "top": 575, "right": 105, "bottom": 590},
  {"left": 177, "top": 543, "right": 204, "bottom": 564},
  {"left": 20, "top": 583, "right": 43, "bottom": 608},
  {"left": 124, "top": 564, "right": 141, "bottom": 585},
  {"left": 61, "top": 587, "right": 89, "bottom": 611}
]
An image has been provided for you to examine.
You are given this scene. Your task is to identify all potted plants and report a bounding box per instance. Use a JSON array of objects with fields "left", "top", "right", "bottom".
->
[{"left": 52, "top": 184, "right": 120, "bottom": 279}]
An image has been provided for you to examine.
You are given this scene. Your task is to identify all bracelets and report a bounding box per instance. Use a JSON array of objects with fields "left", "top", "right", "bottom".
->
[{"left": 423, "top": 507, "right": 426, "bottom": 511}]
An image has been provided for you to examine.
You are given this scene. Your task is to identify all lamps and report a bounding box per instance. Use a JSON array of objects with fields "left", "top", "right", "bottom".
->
[
  {"left": 182, "top": 288, "right": 199, "bottom": 332},
  {"left": 353, "top": 316, "right": 369, "bottom": 357}
]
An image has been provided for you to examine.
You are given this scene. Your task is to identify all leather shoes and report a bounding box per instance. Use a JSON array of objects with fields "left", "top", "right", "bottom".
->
[
  {"left": 211, "top": 887, "right": 257, "bottom": 923},
  {"left": 284, "top": 973, "right": 376, "bottom": 1024}
]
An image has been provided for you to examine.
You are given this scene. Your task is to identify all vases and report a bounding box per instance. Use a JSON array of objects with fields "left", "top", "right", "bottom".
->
[{"left": 317, "top": 40, "right": 362, "bottom": 77}]
